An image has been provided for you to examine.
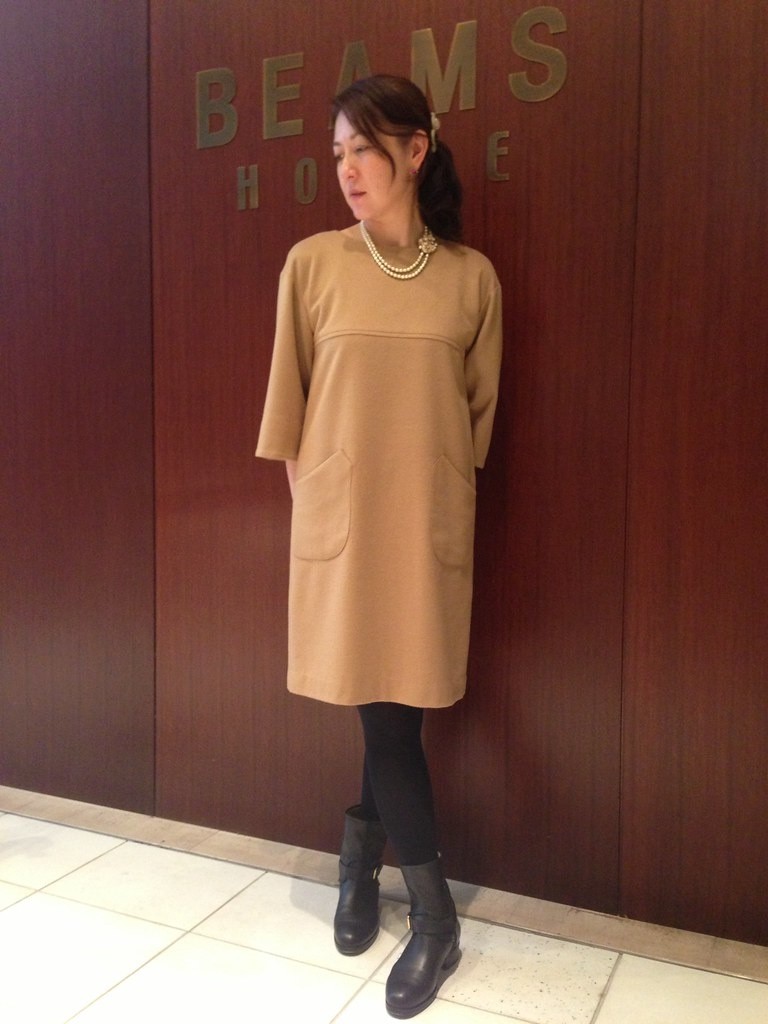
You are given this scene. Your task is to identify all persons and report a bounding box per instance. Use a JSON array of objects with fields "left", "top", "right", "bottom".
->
[{"left": 253, "top": 75, "right": 506, "bottom": 1017}]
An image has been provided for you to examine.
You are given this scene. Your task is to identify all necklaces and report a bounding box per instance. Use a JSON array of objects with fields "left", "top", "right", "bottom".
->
[{"left": 360, "top": 213, "right": 437, "bottom": 280}]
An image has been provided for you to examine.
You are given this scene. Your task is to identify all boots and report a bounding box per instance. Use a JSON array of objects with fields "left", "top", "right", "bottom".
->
[
  {"left": 333, "top": 803, "right": 385, "bottom": 957},
  {"left": 386, "top": 851, "right": 462, "bottom": 1019}
]
